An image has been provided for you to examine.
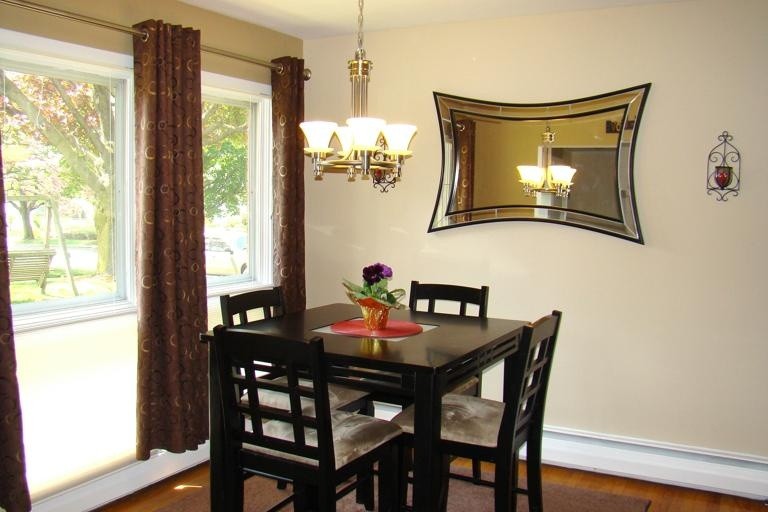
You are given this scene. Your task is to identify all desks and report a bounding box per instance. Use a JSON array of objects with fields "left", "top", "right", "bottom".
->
[{"left": 201, "top": 300, "right": 536, "bottom": 510}]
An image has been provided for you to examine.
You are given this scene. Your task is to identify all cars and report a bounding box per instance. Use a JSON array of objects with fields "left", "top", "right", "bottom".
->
[{"left": 204, "top": 237, "right": 238, "bottom": 277}]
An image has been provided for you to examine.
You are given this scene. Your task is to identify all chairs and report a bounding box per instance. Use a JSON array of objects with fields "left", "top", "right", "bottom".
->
[
  {"left": 382, "top": 307, "right": 563, "bottom": 508},
  {"left": 352, "top": 282, "right": 492, "bottom": 484},
  {"left": 210, "top": 323, "right": 409, "bottom": 510},
  {"left": 216, "top": 283, "right": 382, "bottom": 508}
]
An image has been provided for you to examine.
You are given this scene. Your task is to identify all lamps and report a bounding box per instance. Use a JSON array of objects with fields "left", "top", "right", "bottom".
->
[
  {"left": 517, "top": 121, "right": 577, "bottom": 198},
  {"left": 298, "top": 0, "right": 418, "bottom": 192}
]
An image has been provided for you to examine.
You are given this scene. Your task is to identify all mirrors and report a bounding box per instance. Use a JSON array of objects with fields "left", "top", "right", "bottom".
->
[{"left": 427, "top": 83, "right": 652, "bottom": 245}]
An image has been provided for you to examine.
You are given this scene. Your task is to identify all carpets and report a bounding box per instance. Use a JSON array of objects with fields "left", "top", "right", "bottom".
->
[{"left": 151, "top": 467, "right": 651, "bottom": 512}]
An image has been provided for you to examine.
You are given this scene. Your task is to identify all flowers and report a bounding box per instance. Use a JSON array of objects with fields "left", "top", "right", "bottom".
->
[{"left": 342, "top": 264, "right": 407, "bottom": 311}]
